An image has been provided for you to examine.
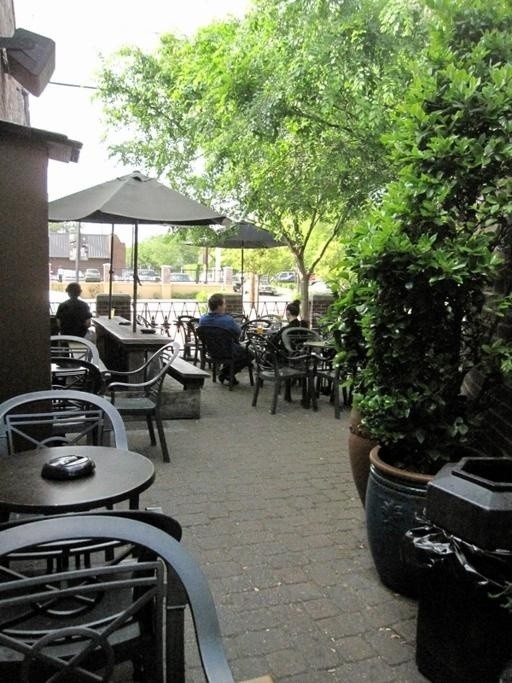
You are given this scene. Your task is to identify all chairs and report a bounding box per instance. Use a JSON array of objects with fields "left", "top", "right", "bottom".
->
[
  {"left": 50, "top": 334, "right": 180, "bottom": 463},
  {"left": 178, "top": 314, "right": 348, "bottom": 418}
]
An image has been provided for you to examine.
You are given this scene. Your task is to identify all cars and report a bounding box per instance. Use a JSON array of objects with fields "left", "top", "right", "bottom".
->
[
  {"left": 84, "top": 264, "right": 190, "bottom": 281},
  {"left": 279, "top": 268, "right": 297, "bottom": 282},
  {"left": 258, "top": 282, "right": 272, "bottom": 295}
]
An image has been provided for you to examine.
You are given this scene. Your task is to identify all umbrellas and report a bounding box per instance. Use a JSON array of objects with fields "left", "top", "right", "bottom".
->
[
  {"left": 46, "top": 172, "right": 231, "bottom": 332},
  {"left": 183, "top": 220, "right": 299, "bottom": 294}
]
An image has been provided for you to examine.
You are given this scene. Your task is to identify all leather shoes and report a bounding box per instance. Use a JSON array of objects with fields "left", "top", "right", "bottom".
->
[
  {"left": 218, "top": 374, "right": 224, "bottom": 383},
  {"left": 225, "top": 376, "right": 238, "bottom": 385}
]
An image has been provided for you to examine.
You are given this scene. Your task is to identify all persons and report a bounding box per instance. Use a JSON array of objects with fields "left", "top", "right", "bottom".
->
[
  {"left": 259, "top": 298, "right": 307, "bottom": 388},
  {"left": 198, "top": 293, "right": 256, "bottom": 384},
  {"left": 56, "top": 282, "right": 98, "bottom": 344}
]
